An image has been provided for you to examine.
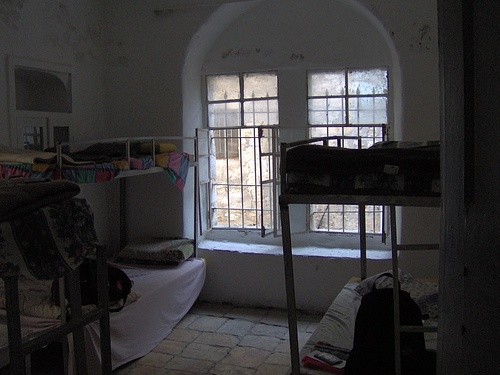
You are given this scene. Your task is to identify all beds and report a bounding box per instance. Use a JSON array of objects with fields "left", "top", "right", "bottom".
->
[
  {"left": 279, "top": 136, "right": 441, "bottom": 375},
  {"left": 0, "top": 128, "right": 206, "bottom": 375}
]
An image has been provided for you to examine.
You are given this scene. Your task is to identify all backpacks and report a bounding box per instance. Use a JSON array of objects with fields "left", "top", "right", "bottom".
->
[
  {"left": 342, "top": 273, "right": 427, "bottom": 375},
  {"left": 51, "top": 259, "right": 134, "bottom": 312}
]
